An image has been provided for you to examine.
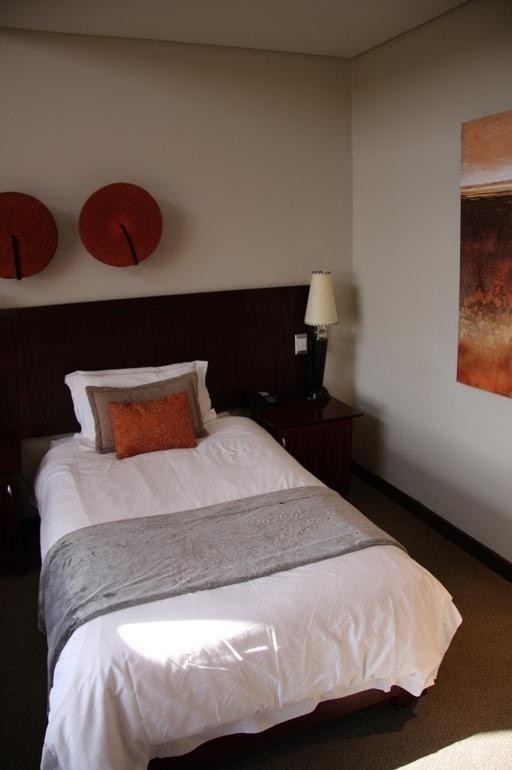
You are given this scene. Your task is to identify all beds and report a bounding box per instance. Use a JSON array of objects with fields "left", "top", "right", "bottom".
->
[{"left": 34, "top": 419, "right": 465, "bottom": 768}]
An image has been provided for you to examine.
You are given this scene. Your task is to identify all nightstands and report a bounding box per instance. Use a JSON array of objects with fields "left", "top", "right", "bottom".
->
[{"left": 257, "top": 392, "right": 365, "bottom": 498}]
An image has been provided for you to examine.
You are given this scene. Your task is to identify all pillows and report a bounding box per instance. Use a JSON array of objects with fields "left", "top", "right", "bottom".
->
[{"left": 63, "top": 361, "right": 220, "bottom": 461}]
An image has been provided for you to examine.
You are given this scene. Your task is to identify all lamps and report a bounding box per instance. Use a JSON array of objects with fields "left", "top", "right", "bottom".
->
[{"left": 302, "top": 272, "right": 339, "bottom": 402}]
